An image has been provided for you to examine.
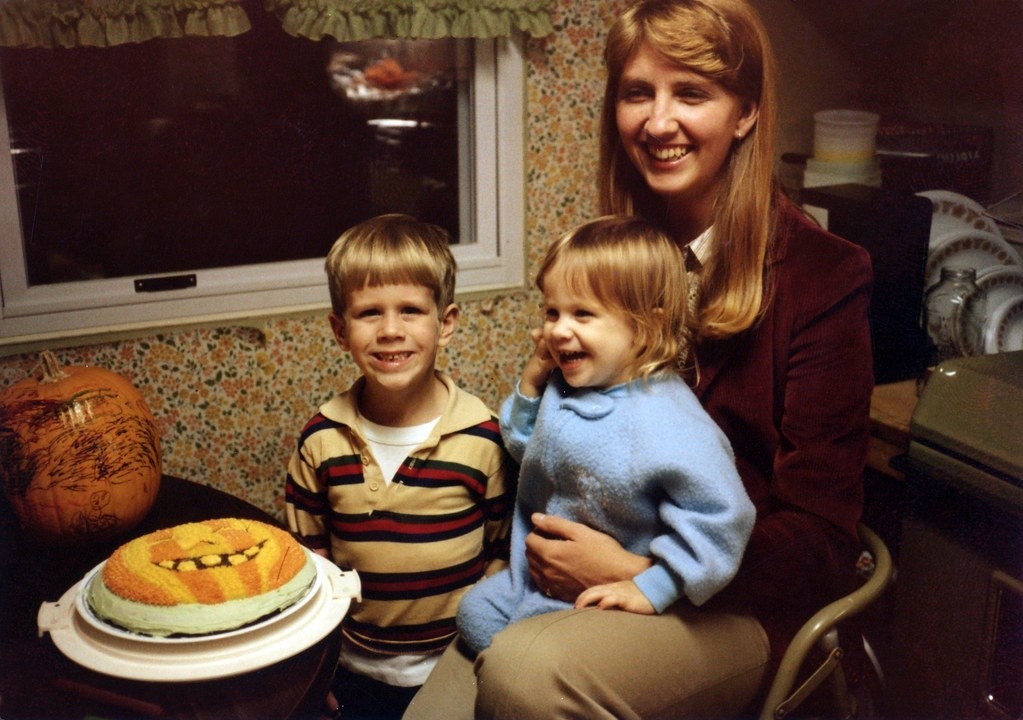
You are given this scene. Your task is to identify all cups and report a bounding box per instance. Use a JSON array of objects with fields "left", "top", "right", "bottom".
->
[{"left": 813, "top": 107, "right": 878, "bottom": 161}]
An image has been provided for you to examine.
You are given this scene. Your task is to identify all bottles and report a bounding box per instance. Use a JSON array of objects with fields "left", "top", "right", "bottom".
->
[{"left": 917, "top": 266, "right": 987, "bottom": 399}]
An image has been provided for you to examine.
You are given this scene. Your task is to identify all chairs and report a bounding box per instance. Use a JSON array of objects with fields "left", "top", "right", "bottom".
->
[{"left": 758, "top": 523, "right": 893, "bottom": 720}]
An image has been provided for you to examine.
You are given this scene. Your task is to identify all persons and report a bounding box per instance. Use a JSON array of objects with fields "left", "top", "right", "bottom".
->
[
  {"left": 454, "top": 215, "right": 757, "bottom": 649},
  {"left": 404, "top": 0, "right": 875, "bottom": 720},
  {"left": 282, "top": 214, "right": 518, "bottom": 720}
]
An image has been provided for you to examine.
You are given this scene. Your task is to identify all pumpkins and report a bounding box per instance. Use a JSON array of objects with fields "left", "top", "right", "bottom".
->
[{"left": 0, "top": 349, "right": 163, "bottom": 545}]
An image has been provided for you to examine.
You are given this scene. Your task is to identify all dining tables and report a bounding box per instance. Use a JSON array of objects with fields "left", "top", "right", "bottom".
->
[{"left": 0, "top": 475, "right": 341, "bottom": 720}]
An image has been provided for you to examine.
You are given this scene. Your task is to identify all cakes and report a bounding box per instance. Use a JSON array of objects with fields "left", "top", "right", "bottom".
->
[{"left": 81, "top": 515, "right": 316, "bottom": 638}]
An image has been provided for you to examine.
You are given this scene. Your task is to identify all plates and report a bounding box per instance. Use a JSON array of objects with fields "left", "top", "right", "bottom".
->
[
  {"left": 74, "top": 545, "right": 317, "bottom": 643},
  {"left": 874, "top": 143, "right": 934, "bottom": 158}
]
